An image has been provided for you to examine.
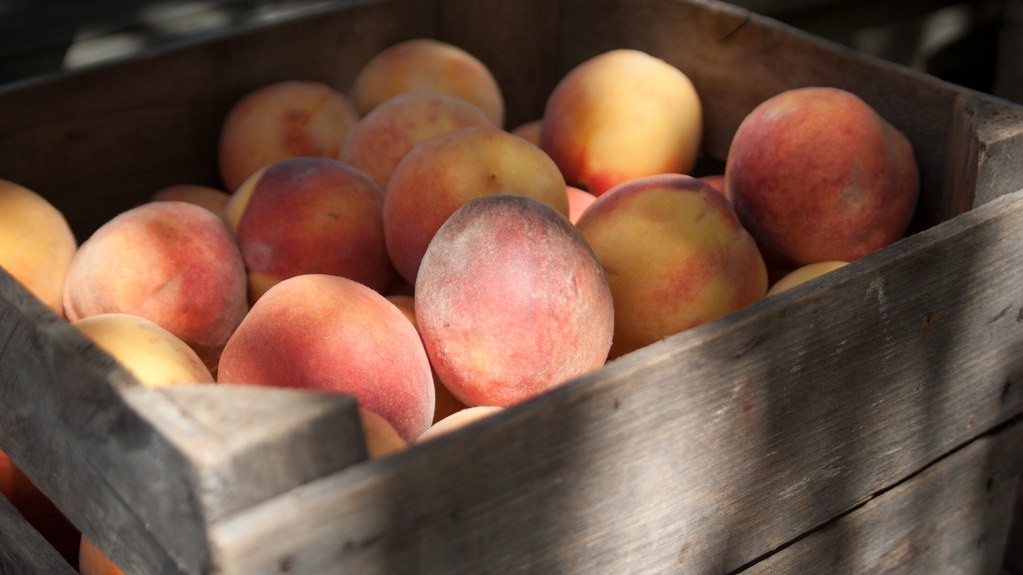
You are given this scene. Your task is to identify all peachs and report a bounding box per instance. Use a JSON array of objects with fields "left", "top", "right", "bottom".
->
[{"left": 0, "top": 34, "right": 919, "bottom": 575}]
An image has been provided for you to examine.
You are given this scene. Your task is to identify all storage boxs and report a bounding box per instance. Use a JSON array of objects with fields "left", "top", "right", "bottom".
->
[{"left": 0, "top": 0, "right": 1023, "bottom": 575}]
